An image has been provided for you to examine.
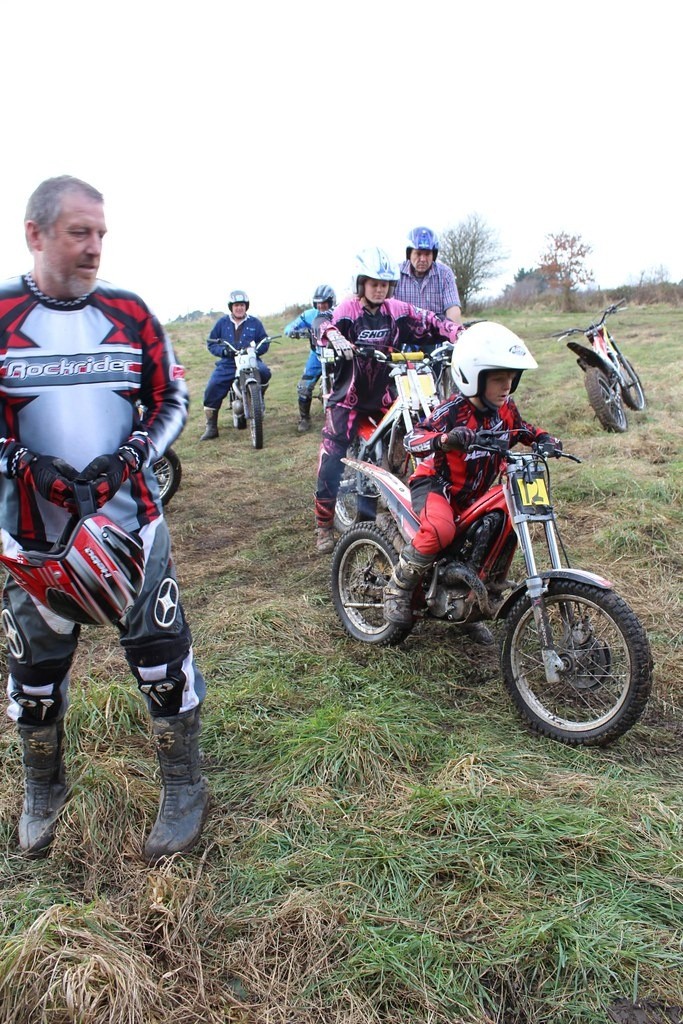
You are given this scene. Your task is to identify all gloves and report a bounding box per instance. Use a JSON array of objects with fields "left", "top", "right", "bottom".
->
[
  {"left": 64, "top": 443, "right": 143, "bottom": 517},
  {"left": 6, "top": 444, "right": 79, "bottom": 509},
  {"left": 222, "top": 347, "right": 236, "bottom": 359},
  {"left": 289, "top": 331, "right": 300, "bottom": 340},
  {"left": 532, "top": 433, "right": 563, "bottom": 460},
  {"left": 325, "top": 330, "right": 360, "bottom": 361},
  {"left": 433, "top": 425, "right": 477, "bottom": 451}
]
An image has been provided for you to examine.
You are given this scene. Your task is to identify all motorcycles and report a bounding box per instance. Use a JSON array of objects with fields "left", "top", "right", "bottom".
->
[
  {"left": 296, "top": 331, "right": 334, "bottom": 416},
  {"left": 334, "top": 341, "right": 454, "bottom": 535},
  {"left": 206, "top": 335, "right": 283, "bottom": 448},
  {"left": 328, "top": 429, "right": 657, "bottom": 748}
]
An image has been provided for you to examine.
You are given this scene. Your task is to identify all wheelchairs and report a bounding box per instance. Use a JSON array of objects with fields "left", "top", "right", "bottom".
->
[{"left": 550, "top": 297, "right": 646, "bottom": 433}]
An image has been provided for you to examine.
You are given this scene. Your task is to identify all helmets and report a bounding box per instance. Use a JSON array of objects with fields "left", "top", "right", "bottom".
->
[
  {"left": 349, "top": 245, "right": 402, "bottom": 295},
  {"left": 228, "top": 290, "right": 249, "bottom": 312},
  {"left": 311, "top": 285, "right": 337, "bottom": 310},
  {"left": 403, "top": 226, "right": 440, "bottom": 262},
  {"left": 450, "top": 321, "right": 539, "bottom": 398},
  {"left": 0, "top": 513, "right": 145, "bottom": 627}
]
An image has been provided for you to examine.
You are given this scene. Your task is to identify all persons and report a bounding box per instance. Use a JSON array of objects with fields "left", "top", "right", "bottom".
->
[
  {"left": 285, "top": 284, "right": 337, "bottom": 436},
  {"left": 393, "top": 226, "right": 464, "bottom": 354},
  {"left": 314, "top": 250, "right": 464, "bottom": 555},
  {"left": 0, "top": 175, "right": 215, "bottom": 866},
  {"left": 382, "top": 322, "right": 562, "bottom": 648},
  {"left": 200, "top": 290, "right": 271, "bottom": 442}
]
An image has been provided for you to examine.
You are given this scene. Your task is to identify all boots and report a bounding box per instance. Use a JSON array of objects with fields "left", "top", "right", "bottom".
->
[
  {"left": 17, "top": 724, "right": 67, "bottom": 860},
  {"left": 313, "top": 491, "right": 337, "bottom": 554},
  {"left": 259, "top": 383, "right": 270, "bottom": 413},
  {"left": 462, "top": 620, "right": 495, "bottom": 645},
  {"left": 142, "top": 705, "right": 211, "bottom": 865},
  {"left": 200, "top": 406, "right": 219, "bottom": 441},
  {"left": 383, "top": 544, "right": 435, "bottom": 627},
  {"left": 296, "top": 398, "right": 311, "bottom": 432}
]
